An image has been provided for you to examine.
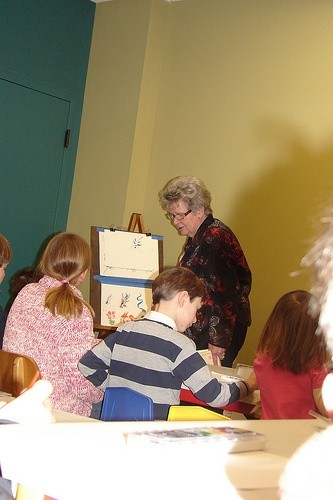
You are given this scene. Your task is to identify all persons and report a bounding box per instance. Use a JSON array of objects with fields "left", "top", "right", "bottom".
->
[
  {"left": 251, "top": 289, "right": 333, "bottom": 420},
  {"left": 160, "top": 174, "right": 251, "bottom": 369},
  {"left": 77, "top": 265, "right": 261, "bottom": 421},
  {"left": 2, "top": 232, "right": 106, "bottom": 416},
  {"left": 0, "top": 232, "right": 12, "bottom": 352}
]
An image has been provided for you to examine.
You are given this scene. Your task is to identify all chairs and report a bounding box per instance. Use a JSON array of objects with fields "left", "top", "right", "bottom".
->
[
  {"left": 0, "top": 350, "right": 43, "bottom": 399},
  {"left": 98, "top": 386, "right": 154, "bottom": 422},
  {"left": 166, "top": 406, "right": 232, "bottom": 422}
]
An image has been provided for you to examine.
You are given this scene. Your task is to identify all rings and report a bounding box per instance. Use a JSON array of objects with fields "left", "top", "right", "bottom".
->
[{"left": 221, "top": 356, "right": 225, "bottom": 359}]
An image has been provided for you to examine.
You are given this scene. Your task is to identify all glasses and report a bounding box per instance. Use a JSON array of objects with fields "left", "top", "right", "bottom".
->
[{"left": 165, "top": 209, "right": 192, "bottom": 220}]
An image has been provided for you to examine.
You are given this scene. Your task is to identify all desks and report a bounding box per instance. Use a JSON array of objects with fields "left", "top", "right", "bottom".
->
[
  {"left": 0, "top": 419, "right": 333, "bottom": 500},
  {"left": 0, "top": 365, "right": 261, "bottom": 425}
]
